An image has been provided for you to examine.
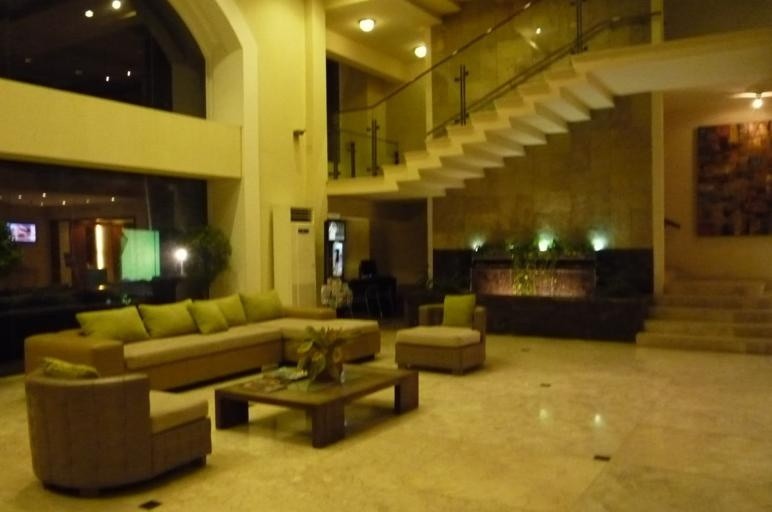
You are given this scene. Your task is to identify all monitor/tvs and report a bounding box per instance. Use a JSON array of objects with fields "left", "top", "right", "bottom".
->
[{"left": 6, "top": 219, "right": 39, "bottom": 244}]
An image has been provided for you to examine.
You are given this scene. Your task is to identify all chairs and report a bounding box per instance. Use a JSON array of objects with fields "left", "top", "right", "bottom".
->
[
  {"left": 343, "top": 277, "right": 398, "bottom": 322},
  {"left": 23, "top": 369, "right": 213, "bottom": 496},
  {"left": 395, "top": 304, "right": 487, "bottom": 376}
]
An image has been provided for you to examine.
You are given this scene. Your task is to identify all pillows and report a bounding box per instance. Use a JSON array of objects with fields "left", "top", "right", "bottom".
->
[
  {"left": 441, "top": 293, "right": 476, "bottom": 327},
  {"left": 74, "top": 289, "right": 287, "bottom": 345},
  {"left": 39, "top": 356, "right": 99, "bottom": 380}
]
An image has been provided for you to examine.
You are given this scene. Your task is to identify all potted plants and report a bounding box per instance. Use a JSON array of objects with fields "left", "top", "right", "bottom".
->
[{"left": 293, "top": 323, "right": 353, "bottom": 390}]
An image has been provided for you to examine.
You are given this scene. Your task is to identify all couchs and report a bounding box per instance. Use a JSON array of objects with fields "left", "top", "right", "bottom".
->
[{"left": 24, "top": 305, "right": 380, "bottom": 391}]
